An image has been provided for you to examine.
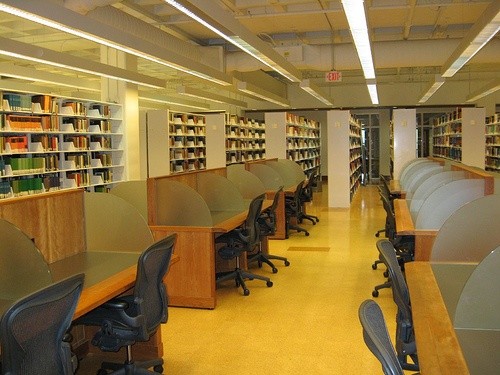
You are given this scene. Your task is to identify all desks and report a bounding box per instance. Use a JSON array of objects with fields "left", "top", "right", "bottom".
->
[
  {"left": 390, "top": 179, "right": 407, "bottom": 199},
  {"left": 404, "top": 259, "right": 500, "bottom": 375},
  {"left": 394, "top": 199, "right": 437, "bottom": 262},
  {"left": 0, "top": 179, "right": 307, "bottom": 360}
]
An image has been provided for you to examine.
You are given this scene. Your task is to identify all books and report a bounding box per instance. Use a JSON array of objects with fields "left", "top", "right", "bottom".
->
[
  {"left": 225, "top": 114, "right": 265, "bottom": 163},
  {"left": 349, "top": 113, "right": 362, "bottom": 202},
  {"left": 0, "top": 90, "right": 113, "bottom": 199},
  {"left": 484, "top": 113, "right": 500, "bottom": 168},
  {"left": 286, "top": 114, "right": 320, "bottom": 176},
  {"left": 169, "top": 112, "right": 207, "bottom": 172},
  {"left": 433, "top": 108, "right": 462, "bottom": 161}
]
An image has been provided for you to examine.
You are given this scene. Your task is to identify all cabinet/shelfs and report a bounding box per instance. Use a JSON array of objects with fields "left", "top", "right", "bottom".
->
[
  {"left": 485, "top": 121, "right": 500, "bottom": 170},
  {"left": 432, "top": 106, "right": 486, "bottom": 169},
  {"left": 390, "top": 109, "right": 416, "bottom": 181},
  {"left": 147, "top": 109, "right": 206, "bottom": 177},
  {"left": 264, "top": 111, "right": 320, "bottom": 177},
  {"left": 0, "top": 88, "right": 129, "bottom": 197},
  {"left": 326, "top": 110, "right": 362, "bottom": 207},
  {"left": 205, "top": 113, "right": 265, "bottom": 170}
]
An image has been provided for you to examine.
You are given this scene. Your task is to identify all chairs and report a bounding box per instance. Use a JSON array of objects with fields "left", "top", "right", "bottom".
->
[
  {"left": 214, "top": 176, "right": 318, "bottom": 296},
  {"left": 0, "top": 272, "right": 85, "bottom": 375},
  {"left": 66, "top": 233, "right": 177, "bottom": 375},
  {"left": 358, "top": 179, "right": 421, "bottom": 375}
]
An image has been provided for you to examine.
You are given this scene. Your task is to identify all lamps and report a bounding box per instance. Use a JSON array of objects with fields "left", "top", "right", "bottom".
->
[{"left": 0, "top": 0, "right": 500, "bottom": 111}]
